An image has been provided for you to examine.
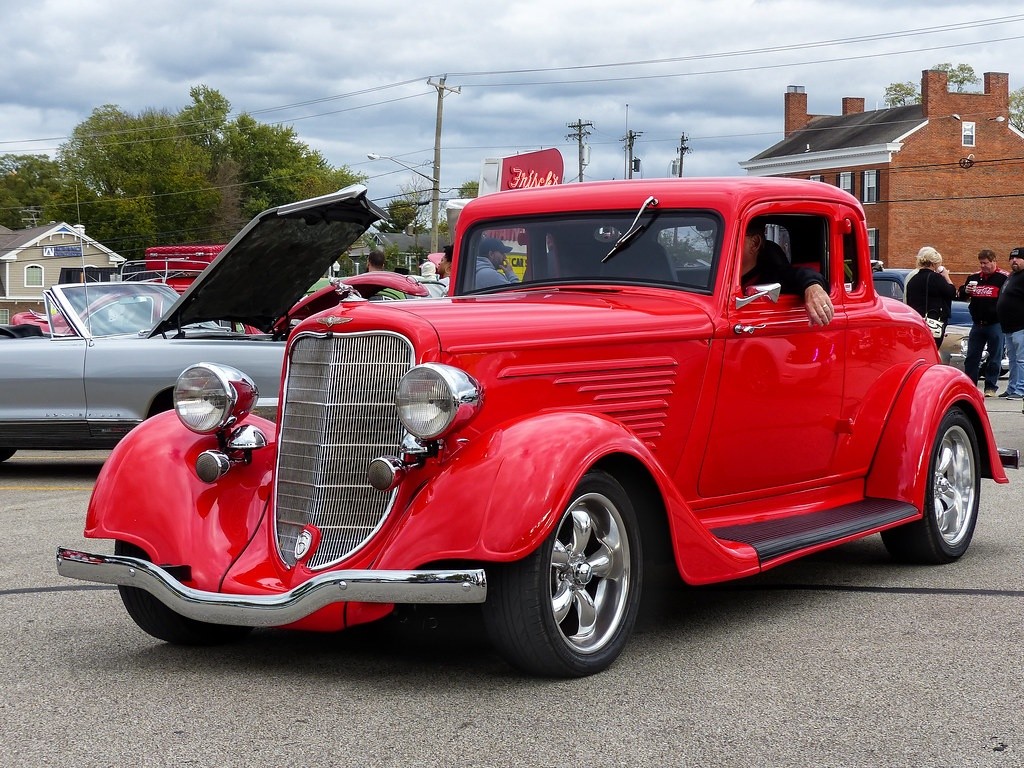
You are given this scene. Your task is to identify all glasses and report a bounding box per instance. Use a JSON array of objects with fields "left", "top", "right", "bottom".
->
[{"left": 705, "top": 237, "right": 714, "bottom": 247}]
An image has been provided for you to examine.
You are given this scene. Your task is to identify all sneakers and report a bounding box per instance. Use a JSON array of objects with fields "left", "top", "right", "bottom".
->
[
  {"left": 984, "top": 390, "right": 995, "bottom": 397},
  {"left": 998, "top": 392, "right": 1008, "bottom": 398},
  {"left": 1006, "top": 393, "right": 1024, "bottom": 400}
]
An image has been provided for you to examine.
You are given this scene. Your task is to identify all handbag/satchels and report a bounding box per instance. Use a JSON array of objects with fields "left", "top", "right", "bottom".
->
[{"left": 922, "top": 309, "right": 945, "bottom": 338}]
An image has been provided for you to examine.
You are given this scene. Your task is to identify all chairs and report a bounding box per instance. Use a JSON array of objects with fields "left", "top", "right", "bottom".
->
[{"left": 625, "top": 241, "right": 677, "bottom": 284}]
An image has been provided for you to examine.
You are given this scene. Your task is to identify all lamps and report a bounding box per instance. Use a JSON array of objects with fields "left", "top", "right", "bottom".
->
[
  {"left": 988, "top": 116, "right": 1005, "bottom": 122},
  {"left": 944, "top": 113, "right": 960, "bottom": 121}
]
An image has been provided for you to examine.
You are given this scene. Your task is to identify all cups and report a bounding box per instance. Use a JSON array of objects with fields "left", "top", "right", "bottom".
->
[
  {"left": 938, "top": 266, "right": 944, "bottom": 273},
  {"left": 969, "top": 281, "right": 978, "bottom": 292}
]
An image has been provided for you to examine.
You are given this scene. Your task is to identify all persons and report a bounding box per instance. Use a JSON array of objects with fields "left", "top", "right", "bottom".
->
[
  {"left": 956, "top": 250, "right": 1010, "bottom": 397},
  {"left": 997, "top": 247, "right": 1024, "bottom": 400},
  {"left": 903, "top": 246, "right": 956, "bottom": 350},
  {"left": 740, "top": 216, "right": 834, "bottom": 327},
  {"left": 475, "top": 238, "right": 520, "bottom": 290},
  {"left": 367, "top": 245, "right": 453, "bottom": 301}
]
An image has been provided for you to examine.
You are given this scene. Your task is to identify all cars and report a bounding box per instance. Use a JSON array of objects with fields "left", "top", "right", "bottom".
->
[
  {"left": 870, "top": 267, "right": 1010, "bottom": 378},
  {"left": 0, "top": 181, "right": 447, "bottom": 465},
  {"left": 54, "top": 176, "right": 1020, "bottom": 685}
]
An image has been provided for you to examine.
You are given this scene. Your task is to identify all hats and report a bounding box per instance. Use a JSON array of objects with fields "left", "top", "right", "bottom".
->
[
  {"left": 1009, "top": 248, "right": 1024, "bottom": 259},
  {"left": 479, "top": 238, "right": 513, "bottom": 253}
]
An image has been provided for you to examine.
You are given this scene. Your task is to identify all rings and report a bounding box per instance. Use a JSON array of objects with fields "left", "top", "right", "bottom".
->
[{"left": 822, "top": 304, "right": 828, "bottom": 309}]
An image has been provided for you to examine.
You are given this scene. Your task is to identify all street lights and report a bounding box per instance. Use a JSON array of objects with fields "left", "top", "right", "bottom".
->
[{"left": 366, "top": 151, "right": 442, "bottom": 254}]
